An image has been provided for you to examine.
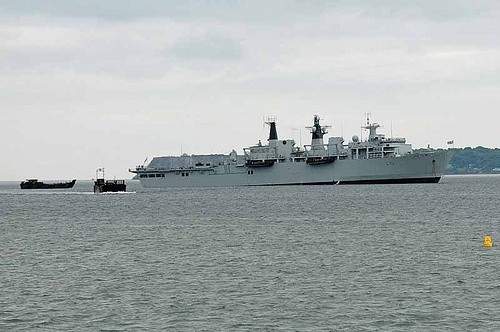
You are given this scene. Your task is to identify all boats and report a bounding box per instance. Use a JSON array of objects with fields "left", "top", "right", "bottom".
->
[
  {"left": 18, "top": 178, "right": 76, "bottom": 190},
  {"left": 91, "top": 167, "right": 127, "bottom": 193}
]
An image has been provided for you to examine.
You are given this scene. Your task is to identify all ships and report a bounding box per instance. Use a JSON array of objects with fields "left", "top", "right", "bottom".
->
[{"left": 125, "top": 109, "right": 455, "bottom": 187}]
